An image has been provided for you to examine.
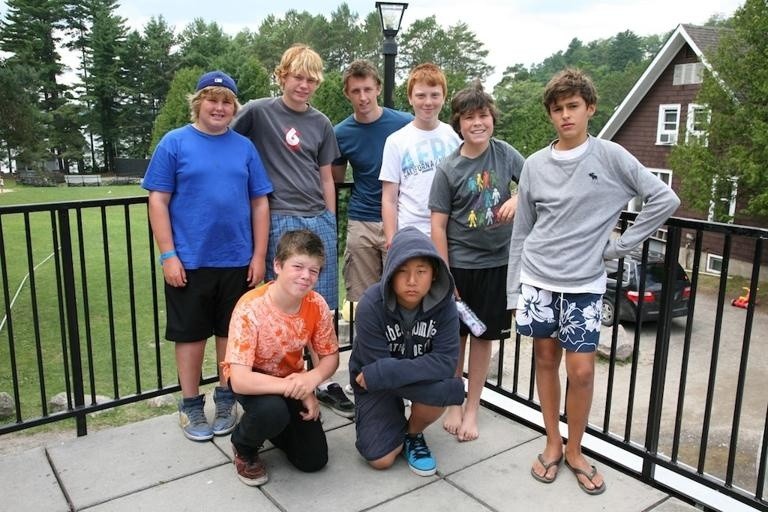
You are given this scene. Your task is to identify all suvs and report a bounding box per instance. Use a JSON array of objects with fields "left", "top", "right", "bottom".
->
[{"left": 596, "top": 248, "right": 693, "bottom": 326}]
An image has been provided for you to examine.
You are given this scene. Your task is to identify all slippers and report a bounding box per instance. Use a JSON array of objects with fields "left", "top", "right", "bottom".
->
[
  {"left": 565, "top": 457, "right": 606, "bottom": 495},
  {"left": 532, "top": 452, "right": 564, "bottom": 483}
]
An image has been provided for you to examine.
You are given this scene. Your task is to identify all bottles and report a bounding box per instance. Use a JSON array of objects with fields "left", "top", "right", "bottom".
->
[{"left": 454, "top": 298, "right": 486, "bottom": 339}]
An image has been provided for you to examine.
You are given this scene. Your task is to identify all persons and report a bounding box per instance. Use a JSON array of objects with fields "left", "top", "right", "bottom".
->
[
  {"left": 225, "top": 228, "right": 340, "bottom": 485},
  {"left": 379, "top": 64, "right": 462, "bottom": 249},
  {"left": 328, "top": 63, "right": 417, "bottom": 408},
  {"left": 428, "top": 83, "right": 525, "bottom": 442},
  {"left": 142, "top": 70, "right": 276, "bottom": 440},
  {"left": 348, "top": 226, "right": 465, "bottom": 474},
  {"left": 229, "top": 44, "right": 359, "bottom": 421},
  {"left": 505, "top": 69, "right": 682, "bottom": 498}
]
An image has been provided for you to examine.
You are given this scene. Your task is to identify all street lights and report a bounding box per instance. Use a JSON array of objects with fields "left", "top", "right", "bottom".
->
[{"left": 372, "top": 1, "right": 409, "bottom": 112}]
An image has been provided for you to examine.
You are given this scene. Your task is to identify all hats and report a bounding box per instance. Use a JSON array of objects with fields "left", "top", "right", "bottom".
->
[{"left": 198, "top": 71, "right": 238, "bottom": 93}]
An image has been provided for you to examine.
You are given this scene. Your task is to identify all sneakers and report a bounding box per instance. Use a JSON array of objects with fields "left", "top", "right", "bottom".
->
[
  {"left": 232, "top": 442, "right": 268, "bottom": 486},
  {"left": 213, "top": 387, "right": 238, "bottom": 435},
  {"left": 178, "top": 394, "right": 213, "bottom": 440},
  {"left": 402, "top": 431, "right": 437, "bottom": 476},
  {"left": 316, "top": 383, "right": 356, "bottom": 418}
]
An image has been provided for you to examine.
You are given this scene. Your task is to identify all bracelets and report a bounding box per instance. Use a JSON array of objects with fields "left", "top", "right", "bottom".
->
[{"left": 157, "top": 247, "right": 179, "bottom": 268}]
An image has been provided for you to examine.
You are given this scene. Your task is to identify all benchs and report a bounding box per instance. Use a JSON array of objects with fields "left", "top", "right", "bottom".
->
[{"left": 64, "top": 174, "right": 102, "bottom": 188}]
